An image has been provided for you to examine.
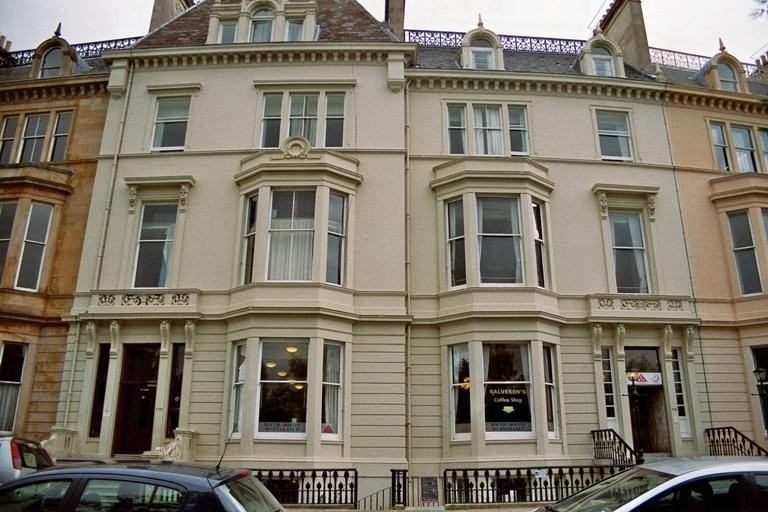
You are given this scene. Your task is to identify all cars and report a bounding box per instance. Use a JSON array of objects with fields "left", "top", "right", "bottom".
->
[
  {"left": 523, "top": 455, "right": 766, "bottom": 512},
  {"left": 0, "top": 437, "right": 56, "bottom": 484},
  {"left": 0, "top": 463, "right": 287, "bottom": 511}
]
